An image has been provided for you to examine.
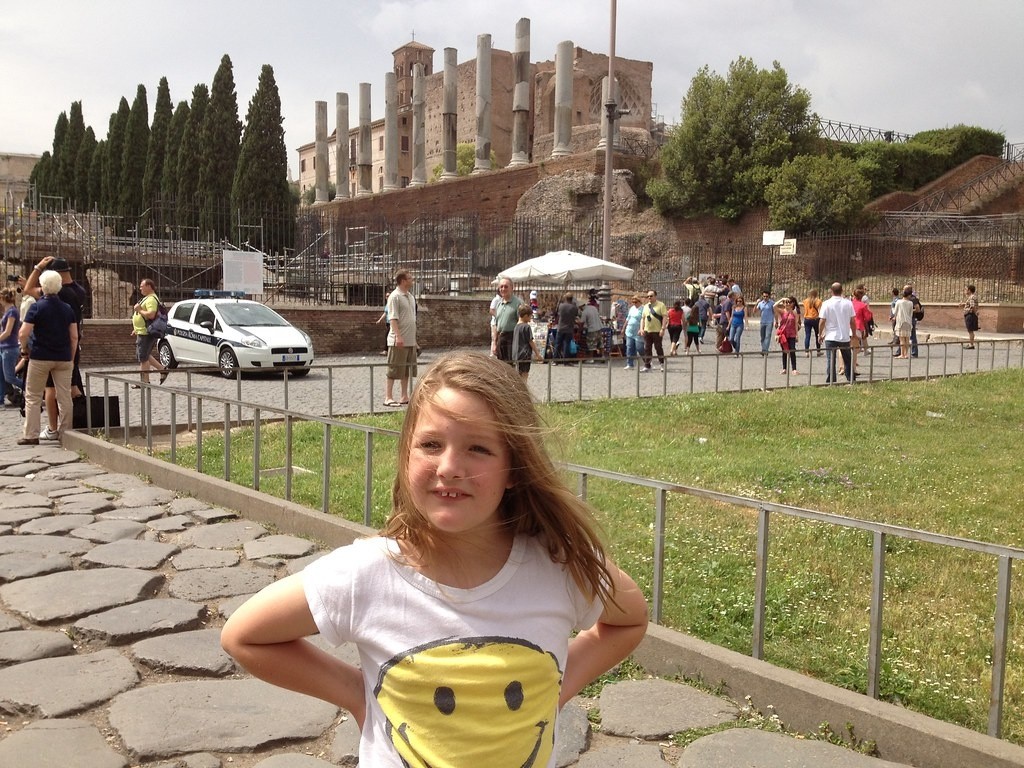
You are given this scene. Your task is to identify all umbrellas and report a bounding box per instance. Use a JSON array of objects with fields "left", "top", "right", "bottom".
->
[{"left": 491, "top": 250, "right": 634, "bottom": 313}]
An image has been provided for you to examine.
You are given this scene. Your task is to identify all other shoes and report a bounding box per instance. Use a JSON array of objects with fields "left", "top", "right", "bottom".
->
[
  {"left": 417, "top": 347, "right": 423, "bottom": 357},
  {"left": 0, "top": 405, "right": 6, "bottom": 411},
  {"left": 550, "top": 339, "right": 974, "bottom": 375},
  {"left": 17, "top": 437, "right": 39, "bottom": 445},
  {"left": 380, "top": 350, "right": 387, "bottom": 355}
]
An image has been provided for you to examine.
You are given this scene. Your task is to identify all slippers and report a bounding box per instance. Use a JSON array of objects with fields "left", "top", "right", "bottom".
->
[
  {"left": 383, "top": 401, "right": 401, "bottom": 407},
  {"left": 401, "top": 400, "right": 411, "bottom": 404}
]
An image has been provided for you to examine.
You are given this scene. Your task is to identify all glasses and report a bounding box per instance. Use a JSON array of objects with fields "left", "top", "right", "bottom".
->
[{"left": 647, "top": 295, "right": 653, "bottom": 297}]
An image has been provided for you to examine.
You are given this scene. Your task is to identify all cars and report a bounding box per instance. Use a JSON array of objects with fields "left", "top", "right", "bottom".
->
[{"left": 154, "top": 288, "right": 314, "bottom": 381}]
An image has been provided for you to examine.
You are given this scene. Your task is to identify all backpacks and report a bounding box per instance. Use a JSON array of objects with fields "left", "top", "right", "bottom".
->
[
  {"left": 139, "top": 296, "right": 167, "bottom": 340},
  {"left": 690, "top": 284, "right": 701, "bottom": 303},
  {"left": 912, "top": 298, "right": 924, "bottom": 322}
]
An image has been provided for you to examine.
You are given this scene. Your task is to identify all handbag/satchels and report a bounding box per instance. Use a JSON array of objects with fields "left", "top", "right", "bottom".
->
[
  {"left": 687, "top": 325, "right": 699, "bottom": 333},
  {"left": 570, "top": 341, "right": 577, "bottom": 354},
  {"left": 718, "top": 337, "right": 733, "bottom": 353},
  {"left": 774, "top": 335, "right": 780, "bottom": 344}
]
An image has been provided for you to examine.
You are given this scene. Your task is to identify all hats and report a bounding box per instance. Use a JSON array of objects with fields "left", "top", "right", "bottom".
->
[{"left": 47, "top": 258, "right": 71, "bottom": 271}]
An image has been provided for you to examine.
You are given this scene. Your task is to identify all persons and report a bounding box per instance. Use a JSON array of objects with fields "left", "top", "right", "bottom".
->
[
  {"left": 130, "top": 278, "right": 169, "bottom": 389},
  {"left": 220, "top": 351, "right": 648, "bottom": 767},
  {"left": 376, "top": 269, "right": 423, "bottom": 407},
  {"left": 0, "top": 256, "right": 86, "bottom": 445},
  {"left": 963, "top": 286, "right": 979, "bottom": 350},
  {"left": 489, "top": 274, "right": 924, "bottom": 383}
]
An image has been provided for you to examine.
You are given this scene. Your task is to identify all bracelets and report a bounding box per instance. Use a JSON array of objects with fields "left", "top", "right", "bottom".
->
[{"left": 34, "top": 265, "right": 43, "bottom": 272}]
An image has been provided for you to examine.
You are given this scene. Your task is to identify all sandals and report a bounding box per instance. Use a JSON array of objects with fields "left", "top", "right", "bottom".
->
[
  {"left": 131, "top": 382, "right": 151, "bottom": 388},
  {"left": 160, "top": 367, "right": 170, "bottom": 385}
]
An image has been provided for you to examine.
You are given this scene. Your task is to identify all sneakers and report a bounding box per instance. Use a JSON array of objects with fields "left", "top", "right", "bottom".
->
[{"left": 39, "top": 425, "right": 58, "bottom": 440}]
